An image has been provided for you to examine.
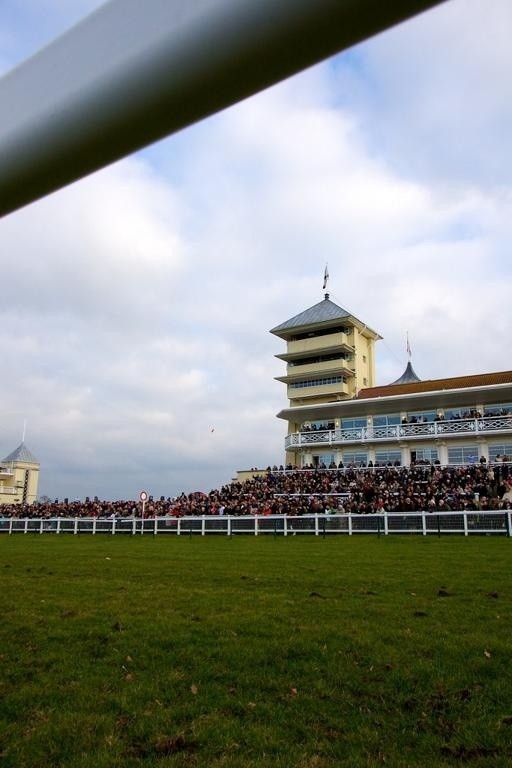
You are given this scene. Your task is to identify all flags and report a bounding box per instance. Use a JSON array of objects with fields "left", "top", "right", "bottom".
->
[
  {"left": 322, "top": 262, "right": 330, "bottom": 290},
  {"left": 406, "top": 338, "right": 413, "bottom": 360}
]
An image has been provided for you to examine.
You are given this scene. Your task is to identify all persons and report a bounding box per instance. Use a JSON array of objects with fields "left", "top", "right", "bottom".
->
[
  {"left": 1, "top": 488, "right": 224, "bottom": 534},
  {"left": 214, "top": 451, "right": 511, "bottom": 533},
  {"left": 296, "top": 404, "right": 512, "bottom": 443}
]
